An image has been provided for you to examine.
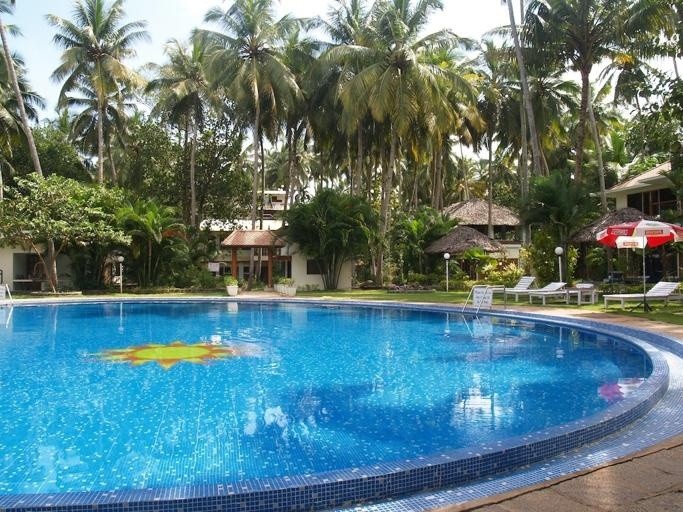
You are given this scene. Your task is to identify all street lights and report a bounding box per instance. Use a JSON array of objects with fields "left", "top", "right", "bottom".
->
[
  {"left": 117, "top": 256, "right": 124, "bottom": 294},
  {"left": 554, "top": 247, "right": 563, "bottom": 291},
  {"left": 444, "top": 252, "right": 450, "bottom": 292}
]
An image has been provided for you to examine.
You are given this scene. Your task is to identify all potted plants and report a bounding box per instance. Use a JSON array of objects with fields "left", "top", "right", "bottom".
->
[
  {"left": 273, "top": 276, "right": 297, "bottom": 298},
  {"left": 223, "top": 275, "right": 239, "bottom": 295}
]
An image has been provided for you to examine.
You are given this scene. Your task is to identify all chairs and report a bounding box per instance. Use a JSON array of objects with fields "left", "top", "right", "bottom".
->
[
  {"left": 603, "top": 280, "right": 680, "bottom": 311},
  {"left": 493, "top": 276, "right": 598, "bottom": 305}
]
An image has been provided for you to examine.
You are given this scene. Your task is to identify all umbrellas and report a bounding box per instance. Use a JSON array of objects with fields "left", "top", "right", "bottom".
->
[
  {"left": 424, "top": 225, "right": 505, "bottom": 253},
  {"left": 571, "top": 207, "right": 658, "bottom": 242},
  {"left": 596, "top": 216, "right": 683, "bottom": 311},
  {"left": 435, "top": 196, "right": 524, "bottom": 226}
]
